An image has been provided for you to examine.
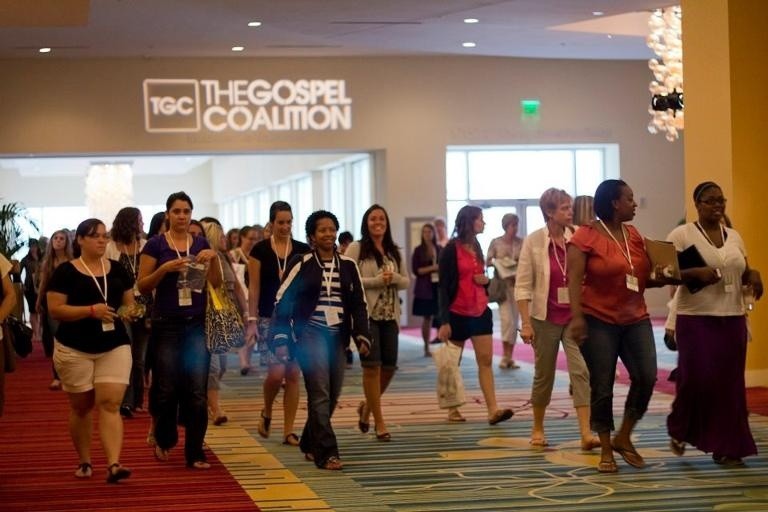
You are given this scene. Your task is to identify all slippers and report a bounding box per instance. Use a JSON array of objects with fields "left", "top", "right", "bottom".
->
[
  {"left": 487, "top": 408, "right": 513, "bottom": 425},
  {"left": 609, "top": 432, "right": 646, "bottom": 469},
  {"left": 581, "top": 436, "right": 600, "bottom": 450},
  {"left": 598, "top": 453, "right": 619, "bottom": 472},
  {"left": 153, "top": 442, "right": 170, "bottom": 462},
  {"left": 447, "top": 408, "right": 467, "bottom": 421},
  {"left": 191, "top": 458, "right": 211, "bottom": 471},
  {"left": 669, "top": 431, "right": 687, "bottom": 457},
  {"left": 281, "top": 432, "right": 300, "bottom": 445},
  {"left": 321, "top": 456, "right": 341, "bottom": 469},
  {"left": 529, "top": 433, "right": 548, "bottom": 447},
  {"left": 104, "top": 461, "right": 132, "bottom": 483},
  {"left": 711, "top": 448, "right": 744, "bottom": 466},
  {"left": 73, "top": 462, "right": 94, "bottom": 477},
  {"left": 257, "top": 407, "right": 272, "bottom": 439}
]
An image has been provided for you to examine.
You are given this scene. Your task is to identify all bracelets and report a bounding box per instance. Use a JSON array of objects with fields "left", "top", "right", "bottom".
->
[
  {"left": 521, "top": 319, "right": 531, "bottom": 328},
  {"left": 90, "top": 305, "right": 102, "bottom": 321},
  {"left": 242, "top": 311, "right": 249, "bottom": 317},
  {"left": 248, "top": 316, "right": 259, "bottom": 322}
]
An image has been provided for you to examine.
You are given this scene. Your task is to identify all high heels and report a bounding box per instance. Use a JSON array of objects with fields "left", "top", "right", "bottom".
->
[{"left": 498, "top": 354, "right": 517, "bottom": 371}]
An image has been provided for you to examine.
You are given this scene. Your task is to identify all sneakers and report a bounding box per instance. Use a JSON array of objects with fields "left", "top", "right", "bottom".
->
[{"left": 209, "top": 410, "right": 228, "bottom": 428}]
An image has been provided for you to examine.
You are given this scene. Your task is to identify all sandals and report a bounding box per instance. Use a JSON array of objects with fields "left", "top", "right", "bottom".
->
[
  {"left": 356, "top": 398, "right": 372, "bottom": 433},
  {"left": 372, "top": 420, "right": 390, "bottom": 442}
]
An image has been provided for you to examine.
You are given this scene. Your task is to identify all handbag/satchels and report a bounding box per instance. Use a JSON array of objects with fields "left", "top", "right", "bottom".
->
[
  {"left": 430, "top": 337, "right": 467, "bottom": 410},
  {"left": 6, "top": 314, "right": 35, "bottom": 358},
  {"left": 487, "top": 264, "right": 508, "bottom": 304},
  {"left": 204, "top": 248, "right": 245, "bottom": 355}
]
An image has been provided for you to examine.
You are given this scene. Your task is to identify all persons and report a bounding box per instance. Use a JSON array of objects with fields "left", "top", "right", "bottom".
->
[
  {"left": 430, "top": 218, "right": 452, "bottom": 344},
  {"left": 226, "top": 225, "right": 257, "bottom": 373},
  {"left": 147, "top": 218, "right": 213, "bottom": 452},
  {"left": 46, "top": 218, "right": 135, "bottom": 484},
  {"left": 513, "top": 188, "right": 605, "bottom": 451},
  {"left": 273, "top": 211, "right": 372, "bottom": 469},
  {"left": 247, "top": 200, "right": 312, "bottom": 446},
  {"left": 487, "top": 214, "right": 527, "bottom": 370},
  {"left": 337, "top": 231, "right": 366, "bottom": 368},
  {"left": 665, "top": 182, "right": 765, "bottom": 465},
  {"left": 568, "top": 179, "right": 668, "bottom": 470},
  {"left": 104, "top": 207, "right": 146, "bottom": 418},
  {"left": 137, "top": 191, "right": 221, "bottom": 470},
  {"left": 202, "top": 222, "right": 250, "bottom": 425},
  {"left": 21, "top": 226, "right": 75, "bottom": 391},
  {"left": 146, "top": 211, "right": 170, "bottom": 236},
  {"left": 663, "top": 213, "right": 754, "bottom": 349},
  {"left": 412, "top": 223, "right": 443, "bottom": 358},
  {"left": 344, "top": 205, "right": 410, "bottom": 441},
  {"left": 0, "top": 251, "right": 17, "bottom": 419},
  {"left": 338, "top": 231, "right": 354, "bottom": 367},
  {"left": 436, "top": 205, "right": 515, "bottom": 425}
]
